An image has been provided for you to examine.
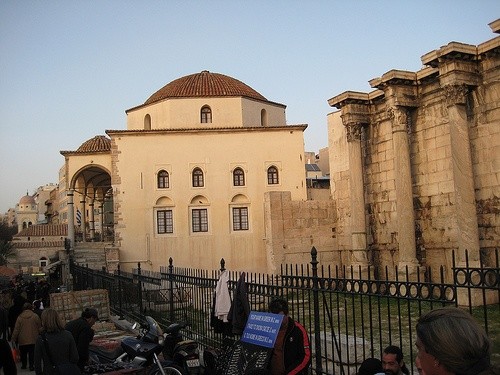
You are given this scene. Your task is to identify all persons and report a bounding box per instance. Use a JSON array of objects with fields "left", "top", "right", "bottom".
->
[
  {"left": 267, "top": 299, "right": 311, "bottom": 375},
  {"left": 358, "top": 308, "right": 499, "bottom": 375},
  {"left": 0, "top": 267, "right": 99, "bottom": 375}
]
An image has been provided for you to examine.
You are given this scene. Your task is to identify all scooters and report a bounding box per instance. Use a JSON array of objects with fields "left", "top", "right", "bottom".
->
[{"left": 86, "top": 314, "right": 201, "bottom": 375}]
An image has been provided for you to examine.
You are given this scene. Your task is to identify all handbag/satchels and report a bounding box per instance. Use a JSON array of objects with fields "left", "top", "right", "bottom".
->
[{"left": 10, "top": 340, "right": 21, "bottom": 363}]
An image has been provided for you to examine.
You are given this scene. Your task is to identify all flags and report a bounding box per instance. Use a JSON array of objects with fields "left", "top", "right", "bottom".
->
[{"left": 76, "top": 209, "right": 81, "bottom": 227}]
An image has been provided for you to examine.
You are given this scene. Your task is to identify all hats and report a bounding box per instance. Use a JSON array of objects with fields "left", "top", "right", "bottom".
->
[{"left": 84, "top": 308, "right": 100, "bottom": 321}]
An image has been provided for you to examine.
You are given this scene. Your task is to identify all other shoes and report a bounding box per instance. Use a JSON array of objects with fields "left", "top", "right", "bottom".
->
[
  {"left": 28, "top": 367, "right": 34, "bottom": 371},
  {"left": 21, "top": 366, "right": 27, "bottom": 370}
]
